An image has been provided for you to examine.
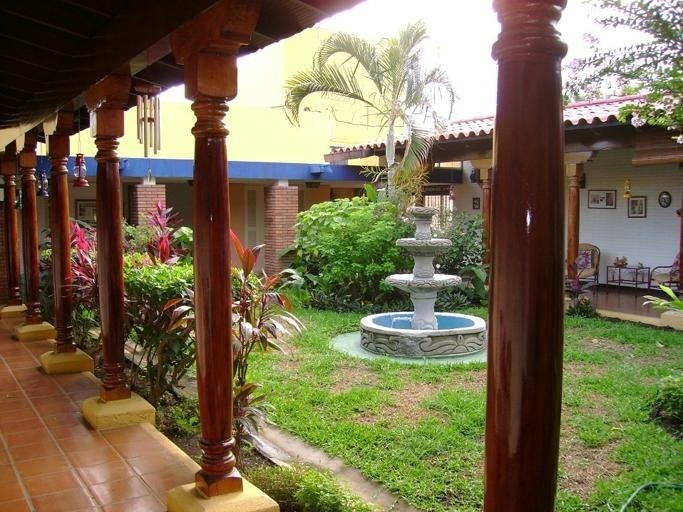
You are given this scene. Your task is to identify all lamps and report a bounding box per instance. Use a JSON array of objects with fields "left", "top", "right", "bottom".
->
[
  {"left": 446, "top": 161, "right": 456, "bottom": 201},
  {"left": 622, "top": 148, "right": 632, "bottom": 201},
  {"left": 12, "top": 156, "right": 23, "bottom": 209},
  {"left": 73, "top": 106, "right": 90, "bottom": 188},
  {"left": 35, "top": 137, "right": 50, "bottom": 198}
]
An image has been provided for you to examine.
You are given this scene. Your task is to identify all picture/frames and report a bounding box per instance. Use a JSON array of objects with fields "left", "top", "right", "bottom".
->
[
  {"left": 471, "top": 197, "right": 480, "bottom": 210},
  {"left": 587, "top": 189, "right": 616, "bottom": 209},
  {"left": 627, "top": 195, "right": 647, "bottom": 218}
]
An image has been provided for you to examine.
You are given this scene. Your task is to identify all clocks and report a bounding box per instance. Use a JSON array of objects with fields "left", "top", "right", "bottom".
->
[{"left": 658, "top": 191, "right": 671, "bottom": 208}]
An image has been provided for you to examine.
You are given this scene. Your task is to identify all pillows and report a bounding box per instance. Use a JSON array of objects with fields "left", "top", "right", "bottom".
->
[{"left": 576, "top": 249, "right": 594, "bottom": 269}]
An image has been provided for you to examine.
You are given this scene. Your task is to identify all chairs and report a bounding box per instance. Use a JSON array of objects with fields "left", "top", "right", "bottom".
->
[
  {"left": 562, "top": 241, "right": 600, "bottom": 294},
  {"left": 648, "top": 248, "right": 680, "bottom": 302}
]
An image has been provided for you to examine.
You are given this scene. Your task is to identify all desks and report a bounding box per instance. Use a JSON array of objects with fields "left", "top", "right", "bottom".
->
[{"left": 606, "top": 264, "right": 650, "bottom": 299}]
